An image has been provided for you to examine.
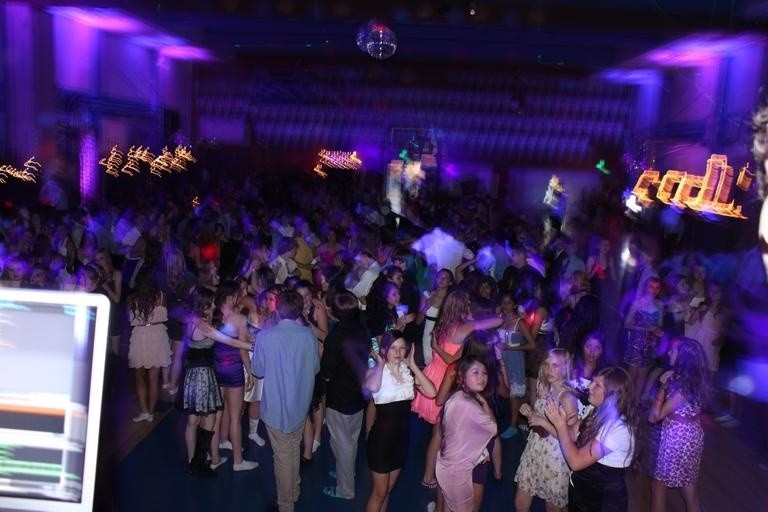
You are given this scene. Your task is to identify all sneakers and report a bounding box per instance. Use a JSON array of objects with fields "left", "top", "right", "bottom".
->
[
  {"left": 328, "top": 471, "right": 336, "bottom": 479},
  {"left": 132, "top": 413, "right": 149, "bottom": 422},
  {"left": 146, "top": 414, "right": 153, "bottom": 422},
  {"left": 169, "top": 385, "right": 179, "bottom": 395},
  {"left": 323, "top": 486, "right": 344, "bottom": 498},
  {"left": 162, "top": 383, "right": 170, "bottom": 390},
  {"left": 248, "top": 432, "right": 265, "bottom": 446},
  {"left": 210, "top": 457, "right": 228, "bottom": 469},
  {"left": 233, "top": 459, "right": 259, "bottom": 471},
  {"left": 502, "top": 426, "right": 518, "bottom": 438},
  {"left": 219, "top": 440, "right": 244, "bottom": 452}
]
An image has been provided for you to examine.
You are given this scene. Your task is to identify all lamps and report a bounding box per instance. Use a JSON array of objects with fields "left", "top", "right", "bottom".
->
[{"left": 356, "top": 22, "right": 399, "bottom": 60}]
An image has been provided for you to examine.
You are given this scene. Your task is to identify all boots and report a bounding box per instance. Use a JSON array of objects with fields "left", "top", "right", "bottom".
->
[{"left": 190, "top": 427, "right": 219, "bottom": 478}]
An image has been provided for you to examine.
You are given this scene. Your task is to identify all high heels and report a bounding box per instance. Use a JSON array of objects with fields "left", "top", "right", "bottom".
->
[{"left": 311, "top": 438, "right": 323, "bottom": 454}]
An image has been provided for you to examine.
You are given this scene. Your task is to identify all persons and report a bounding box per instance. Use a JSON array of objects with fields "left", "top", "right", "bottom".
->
[
  {"left": 623, "top": 278, "right": 665, "bottom": 407},
  {"left": 470, "top": 275, "right": 503, "bottom": 321},
  {"left": 434, "top": 356, "right": 501, "bottom": 512},
  {"left": 367, "top": 265, "right": 404, "bottom": 302},
  {"left": 368, "top": 283, "right": 415, "bottom": 344},
  {"left": 541, "top": 367, "right": 639, "bottom": 512},
  {"left": 252, "top": 290, "right": 321, "bottom": 512},
  {"left": 109, "top": 174, "right": 768, "bottom": 292},
  {"left": 496, "top": 266, "right": 518, "bottom": 344},
  {"left": 519, "top": 277, "right": 555, "bottom": 405},
  {"left": 513, "top": 347, "right": 578, "bottom": 512},
  {"left": 126, "top": 272, "right": 174, "bottom": 422},
  {"left": 642, "top": 273, "right": 689, "bottom": 403},
  {"left": 647, "top": 337, "right": 715, "bottom": 512},
  {"left": 177, "top": 286, "right": 255, "bottom": 473},
  {"left": 213, "top": 281, "right": 260, "bottom": 471},
  {"left": 0, "top": 194, "right": 123, "bottom": 356},
  {"left": 566, "top": 269, "right": 601, "bottom": 355},
  {"left": 415, "top": 269, "right": 458, "bottom": 367},
  {"left": 436, "top": 330, "right": 509, "bottom": 511},
  {"left": 689, "top": 280, "right": 727, "bottom": 411},
  {"left": 318, "top": 288, "right": 368, "bottom": 500},
  {"left": 568, "top": 334, "right": 604, "bottom": 425},
  {"left": 411, "top": 290, "right": 505, "bottom": 487},
  {"left": 363, "top": 330, "right": 437, "bottom": 511}
]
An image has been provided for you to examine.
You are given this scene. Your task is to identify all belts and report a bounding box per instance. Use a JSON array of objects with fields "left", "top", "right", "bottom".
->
[{"left": 133, "top": 321, "right": 164, "bottom": 326}]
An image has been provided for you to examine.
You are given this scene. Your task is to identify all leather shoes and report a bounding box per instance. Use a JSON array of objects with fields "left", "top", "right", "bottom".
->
[{"left": 300, "top": 454, "right": 315, "bottom": 465}]
